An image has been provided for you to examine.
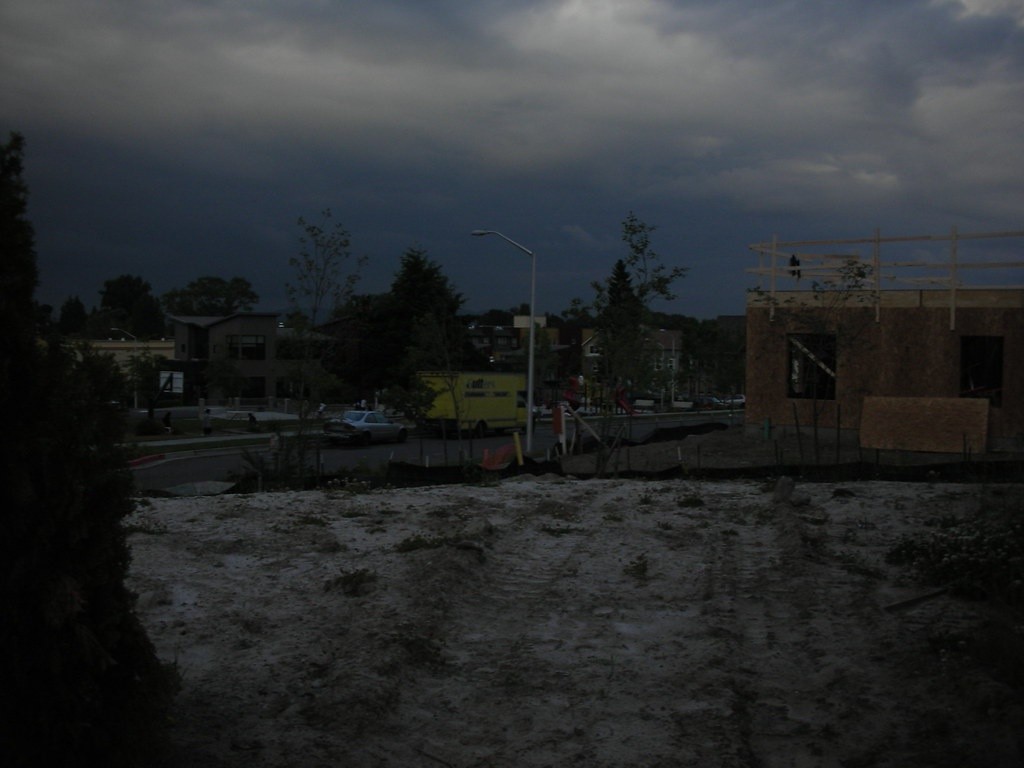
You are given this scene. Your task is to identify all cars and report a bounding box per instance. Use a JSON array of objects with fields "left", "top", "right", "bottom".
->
[
  {"left": 321, "top": 410, "right": 409, "bottom": 448},
  {"left": 694, "top": 393, "right": 745, "bottom": 410}
]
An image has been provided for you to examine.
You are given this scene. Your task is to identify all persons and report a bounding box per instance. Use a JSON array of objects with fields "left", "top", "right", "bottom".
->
[
  {"left": 361, "top": 397, "right": 369, "bottom": 411},
  {"left": 246, "top": 413, "right": 257, "bottom": 431},
  {"left": 202, "top": 409, "right": 213, "bottom": 438},
  {"left": 318, "top": 401, "right": 327, "bottom": 416},
  {"left": 162, "top": 410, "right": 177, "bottom": 435}
]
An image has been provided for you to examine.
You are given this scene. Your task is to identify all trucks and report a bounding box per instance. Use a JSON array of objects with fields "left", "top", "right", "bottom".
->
[{"left": 412, "top": 368, "right": 528, "bottom": 441}]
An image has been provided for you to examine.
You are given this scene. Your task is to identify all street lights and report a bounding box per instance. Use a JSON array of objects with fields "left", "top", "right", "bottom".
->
[
  {"left": 470, "top": 229, "right": 540, "bottom": 455},
  {"left": 643, "top": 337, "right": 665, "bottom": 409},
  {"left": 110, "top": 327, "right": 139, "bottom": 410},
  {"left": 658, "top": 327, "right": 675, "bottom": 407}
]
